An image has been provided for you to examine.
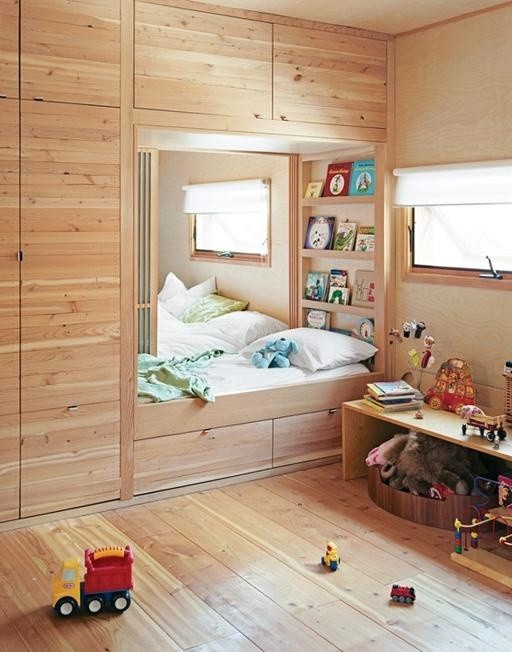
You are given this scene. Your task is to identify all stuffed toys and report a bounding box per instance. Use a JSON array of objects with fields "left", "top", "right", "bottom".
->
[
  {"left": 252, "top": 336, "right": 298, "bottom": 369},
  {"left": 365, "top": 426, "right": 492, "bottom": 499}
]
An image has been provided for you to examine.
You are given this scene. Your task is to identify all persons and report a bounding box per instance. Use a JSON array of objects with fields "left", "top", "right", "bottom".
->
[{"left": 389, "top": 319, "right": 435, "bottom": 371}]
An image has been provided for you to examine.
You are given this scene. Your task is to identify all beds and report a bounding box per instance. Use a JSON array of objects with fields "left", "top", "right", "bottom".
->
[
  {"left": 157, "top": 301, "right": 289, "bottom": 357},
  {"left": 122, "top": 354, "right": 379, "bottom": 501}
]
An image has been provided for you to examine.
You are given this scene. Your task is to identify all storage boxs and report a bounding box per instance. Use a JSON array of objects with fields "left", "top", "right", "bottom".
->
[{"left": 368, "top": 466, "right": 496, "bottom": 532}]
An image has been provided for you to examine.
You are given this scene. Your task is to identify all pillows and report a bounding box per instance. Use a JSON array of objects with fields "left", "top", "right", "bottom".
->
[
  {"left": 164, "top": 276, "right": 216, "bottom": 318},
  {"left": 157, "top": 271, "right": 187, "bottom": 301},
  {"left": 181, "top": 293, "right": 249, "bottom": 324},
  {"left": 241, "top": 325, "right": 377, "bottom": 372},
  {"left": 209, "top": 310, "right": 288, "bottom": 344}
]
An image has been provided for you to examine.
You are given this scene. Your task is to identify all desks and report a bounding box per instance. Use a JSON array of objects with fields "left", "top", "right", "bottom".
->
[{"left": 341, "top": 397, "right": 512, "bottom": 483}]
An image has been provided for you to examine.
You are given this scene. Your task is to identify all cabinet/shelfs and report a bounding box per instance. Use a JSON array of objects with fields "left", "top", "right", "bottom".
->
[
  {"left": 298, "top": 198, "right": 374, "bottom": 317},
  {"left": 134, "top": 373, "right": 384, "bottom": 496},
  {"left": 133, "top": 0, "right": 387, "bottom": 139},
  {"left": 0, "top": 0, "right": 124, "bottom": 109},
  {"left": 0, "top": 99, "right": 124, "bottom": 522}
]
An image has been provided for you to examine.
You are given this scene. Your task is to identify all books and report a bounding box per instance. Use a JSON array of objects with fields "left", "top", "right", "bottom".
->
[
  {"left": 304, "top": 182, "right": 323, "bottom": 198},
  {"left": 363, "top": 382, "right": 420, "bottom": 414},
  {"left": 305, "top": 215, "right": 375, "bottom": 252},
  {"left": 305, "top": 267, "right": 351, "bottom": 306},
  {"left": 322, "top": 161, "right": 353, "bottom": 197},
  {"left": 349, "top": 159, "right": 375, "bottom": 195}
]
